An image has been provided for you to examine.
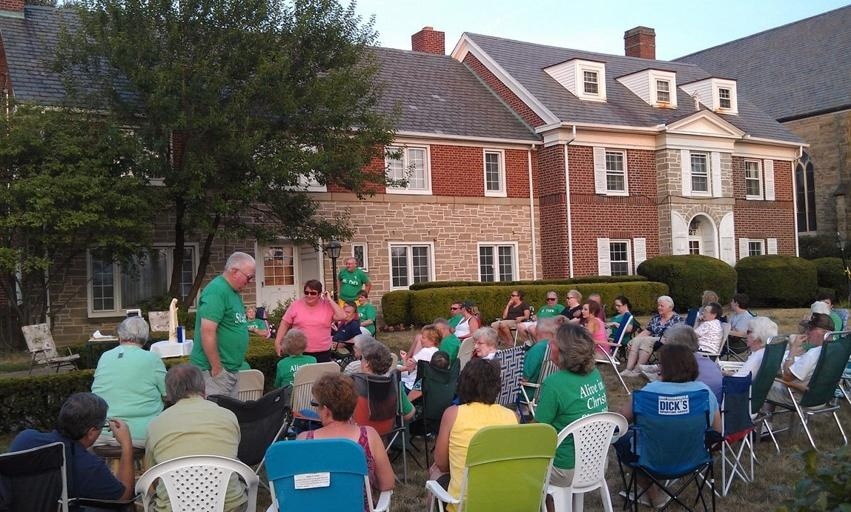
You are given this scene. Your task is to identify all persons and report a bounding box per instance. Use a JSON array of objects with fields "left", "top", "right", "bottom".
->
[
  {"left": 88, "top": 316, "right": 169, "bottom": 449},
  {"left": 246, "top": 307, "right": 270, "bottom": 337},
  {"left": 186, "top": 252, "right": 257, "bottom": 403},
  {"left": 274, "top": 279, "right": 851, "bottom": 512},
  {"left": 145, "top": 364, "right": 247, "bottom": 512},
  {"left": 338, "top": 258, "right": 370, "bottom": 309},
  {"left": 1, "top": 392, "right": 138, "bottom": 512}
]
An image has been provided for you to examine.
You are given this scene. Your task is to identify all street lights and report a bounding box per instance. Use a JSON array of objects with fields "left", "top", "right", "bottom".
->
[{"left": 323, "top": 240, "right": 344, "bottom": 303}]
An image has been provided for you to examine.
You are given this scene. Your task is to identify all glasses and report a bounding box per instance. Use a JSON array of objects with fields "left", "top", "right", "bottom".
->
[
  {"left": 566, "top": 296, "right": 574, "bottom": 299},
  {"left": 304, "top": 291, "right": 318, "bottom": 295},
  {"left": 546, "top": 298, "right": 555, "bottom": 301},
  {"left": 310, "top": 399, "right": 328, "bottom": 408},
  {"left": 88, "top": 420, "right": 110, "bottom": 432},
  {"left": 512, "top": 295, "right": 518, "bottom": 297},
  {"left": 230, "top": 268, "right": 253, "bottom": 282},
  {"left": 451, "top": 307, "right": 460, "bottom": 310}
]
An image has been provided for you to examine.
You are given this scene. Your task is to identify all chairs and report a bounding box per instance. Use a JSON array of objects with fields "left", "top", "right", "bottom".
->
[{"left": 2, "top": 306, "right": 851, "bottom": 512}]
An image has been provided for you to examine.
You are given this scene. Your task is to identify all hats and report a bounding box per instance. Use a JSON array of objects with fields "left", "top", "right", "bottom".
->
[
  {"left": 799, "top": 314, "right": 835, "bottom": 331},
  {"left": 460, "top": 300, "right": 475, "bottom": 309}
]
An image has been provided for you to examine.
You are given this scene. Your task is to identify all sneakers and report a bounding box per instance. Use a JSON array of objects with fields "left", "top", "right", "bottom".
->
[
  {"left": 620, "top": 369, "right": 640, "bottom": 378},
  {"left": 741, "top": 430, "right": 776, "bottom": 442},
  {"left": 619, "top": 489, "right": 673, "bottom": 508},
  {"left": 638, "top": 364, "right": 658, "bottom": 381}
]
